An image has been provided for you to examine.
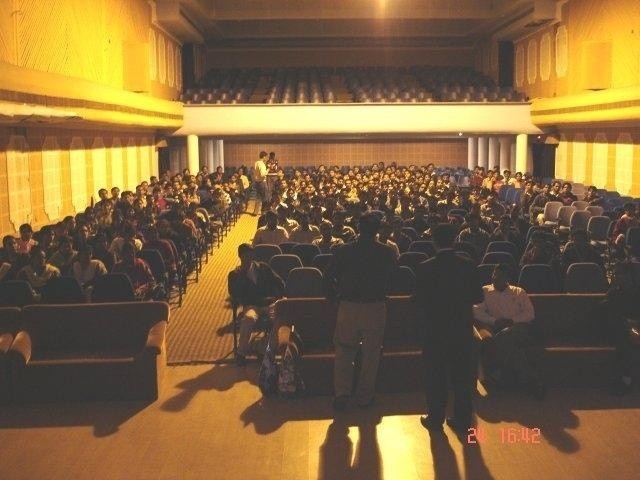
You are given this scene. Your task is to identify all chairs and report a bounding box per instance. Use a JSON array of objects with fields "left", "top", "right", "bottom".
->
[
  {"left": 230, "top": 208, "right": 470, "bottom": 360},
  {"left": 1, "top": 170, "right": 244, "bottom": 300},
  {"left": 181, "top": 66, "right": 529, "bottom": 103},
  {"left": 459, "top": 175, "right": 640, "bottom": 292}
]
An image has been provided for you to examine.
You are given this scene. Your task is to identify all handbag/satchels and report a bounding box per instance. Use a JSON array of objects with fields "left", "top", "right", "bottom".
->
[{"left": 259, "top": 330, "right": 306, "bottom": 399}]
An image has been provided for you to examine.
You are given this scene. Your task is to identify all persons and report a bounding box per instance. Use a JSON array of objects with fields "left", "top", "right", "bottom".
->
[
  {"left": 318, "top": 210, "right": 402, "bottom": 413},
  {"left": 409, "top": 223, "right": 484, "bottom": 433},
  {"left": 0, "top": 148, "right": 639, "bottom": 387}
]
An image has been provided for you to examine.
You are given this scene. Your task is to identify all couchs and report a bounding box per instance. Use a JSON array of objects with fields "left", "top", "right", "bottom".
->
[{"left": 0, "top": 293, "right": 640, "bottom": 403}]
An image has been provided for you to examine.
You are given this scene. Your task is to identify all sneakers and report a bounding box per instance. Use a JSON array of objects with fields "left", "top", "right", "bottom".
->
[{"left": 237, "top": 353, "right": 246, "bottom": 366}]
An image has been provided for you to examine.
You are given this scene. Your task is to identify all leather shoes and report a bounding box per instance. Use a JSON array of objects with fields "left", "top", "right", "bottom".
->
[
  {"left": 421, "top": 415, "right": 443, "bottom": 430},
  {"left": 446, "top": 416, "right": 472, "bottom": 431}
]
